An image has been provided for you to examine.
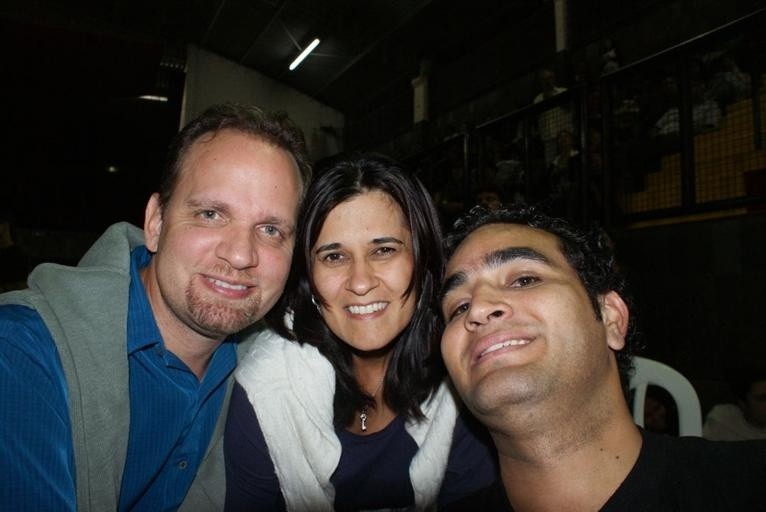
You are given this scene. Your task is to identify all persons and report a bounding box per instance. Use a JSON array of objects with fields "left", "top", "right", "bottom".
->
[
  {"left": 2, "top": 99, "right": 318, "bottom": 511},
  {"left": 416, "top": 199, "right": 766, "bottom": 509},
  {"left": 214, "top": 147, "right": 505, "bottom": 512}
]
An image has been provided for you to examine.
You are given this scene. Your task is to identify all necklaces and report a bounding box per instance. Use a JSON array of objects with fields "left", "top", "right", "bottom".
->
[{"left": 348, "top": 367, "right": 386, "bottom": 434}]
